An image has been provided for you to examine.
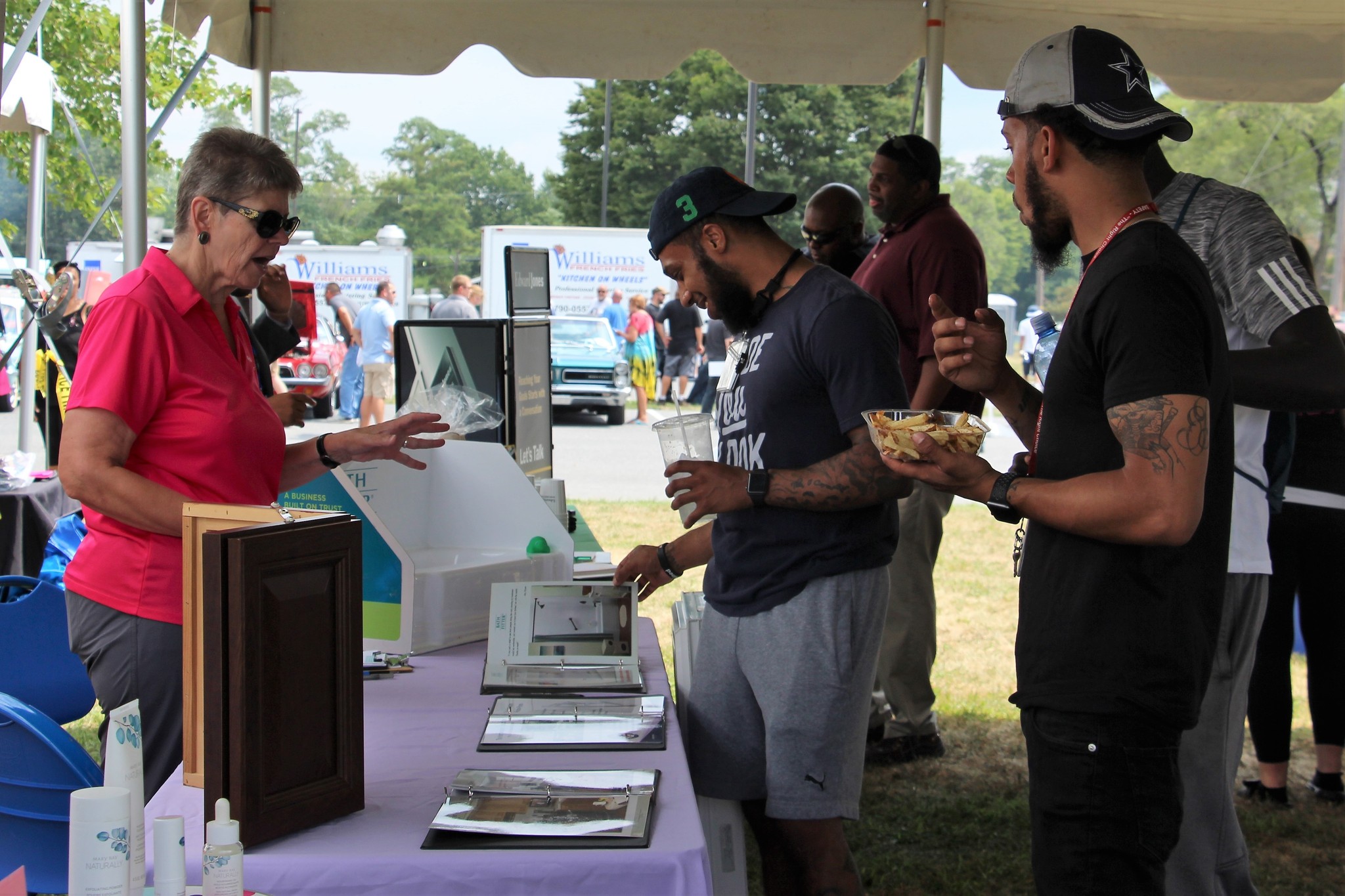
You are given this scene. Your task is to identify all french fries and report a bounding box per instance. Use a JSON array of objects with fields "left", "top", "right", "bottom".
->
[{"left": 869, "top": 411, "right": 985, "bottom": 463}]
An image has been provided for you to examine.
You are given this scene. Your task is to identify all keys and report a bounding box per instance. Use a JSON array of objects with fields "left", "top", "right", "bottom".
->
[{"left": 1013, "top": 547, "right": 1021, "bottom": 577}]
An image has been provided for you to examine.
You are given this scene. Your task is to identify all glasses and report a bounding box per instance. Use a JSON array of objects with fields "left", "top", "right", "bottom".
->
[
  {"left": 207, "top": 195, "right": 300, "bottom": 240},
  {"left": 800, "top": 224, "right": 850, "bottom": 246}
]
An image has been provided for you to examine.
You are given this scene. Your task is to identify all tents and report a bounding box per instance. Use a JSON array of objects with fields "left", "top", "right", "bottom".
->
[{"left": 115, "top": 1, "right": 1344, "bottom": 895}]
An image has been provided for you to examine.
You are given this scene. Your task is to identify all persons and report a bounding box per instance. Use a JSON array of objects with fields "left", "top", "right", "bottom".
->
[
  {"left": 879, "top": 23, "right": 1236, "bottom": 895},
  {"left": 229, "top": 264, "right": 302, "bottom": 397},
  {"left": 1240, "top": 234, "right": 1345, "bottom": 806},
  {"left": 1018, "top": 304, "right": 1044, "bottom": 386},
  {"left": 430, "top": 274, "right": 484, "bottom": 319},
  {"left": 57, "top": 128, "right": 451, "bottom": 810},
  {"left": 610, "top": 165, "right": 918, "bottom": 895},
  {"left": 800, "top": 183, "right": 879, "bottom": 279},
  {"left": 16, "top": 261, "right": 95, "bottom": 466},
  {"left": 324, "top": 280, "right": 398, "bottom": 426},
  {"left": 586, "top": 285, "right": 736, "bottom": 426},
  {"left": 844, "top": 133, "right": 988, "bottom": 766},
  {"left": 1144, "top": 133, "right": 1345, "bottom": 896}
]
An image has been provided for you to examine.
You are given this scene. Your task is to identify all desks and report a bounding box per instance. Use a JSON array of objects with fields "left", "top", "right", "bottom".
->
[
  {"left": 0, "top": 474, "right": 83, "bottom": 576},
  {"left": 144, "top": 504, "right": 714, "bottom": 896}
]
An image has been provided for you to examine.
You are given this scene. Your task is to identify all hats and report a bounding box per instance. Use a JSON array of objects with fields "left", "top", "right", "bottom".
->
[
  {"left": 998, "top": 26, "right": 1193, "bottom": 143},
  {"left": 652, "top": 286, "right": 670, "bottom": 295},
  {"left": 647, "top": 167, "right": 797, "bottom": 261}
]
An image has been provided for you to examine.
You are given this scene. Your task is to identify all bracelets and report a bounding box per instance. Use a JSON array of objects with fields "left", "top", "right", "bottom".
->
[
  {"left": 316, "top": 433, "right": 341, "bottom": 469},
  {"left": 657, "top": 542, "right": 683, "bottom": 579}
]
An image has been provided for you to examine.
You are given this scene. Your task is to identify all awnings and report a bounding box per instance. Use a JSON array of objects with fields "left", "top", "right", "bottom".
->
[{"left": 1, "top": 42, "right": 122, "bottom": 241}]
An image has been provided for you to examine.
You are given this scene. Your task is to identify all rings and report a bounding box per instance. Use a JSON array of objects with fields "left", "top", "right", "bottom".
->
[{"left": 403, "top": 437, "right": 408, "bottom": 448}]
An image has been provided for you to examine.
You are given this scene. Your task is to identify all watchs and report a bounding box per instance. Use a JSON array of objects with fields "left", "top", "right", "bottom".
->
[
  {"left": 987, "top": 472, "right": 1022, "bottom": 524},
  {"left": 746, "top": 468, "right": 770, "bottom": 510}
]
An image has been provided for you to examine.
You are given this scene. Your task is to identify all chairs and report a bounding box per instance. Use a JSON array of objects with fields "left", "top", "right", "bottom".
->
[{"left": 0, "top": 576, "right": 103, "bottom": 894}]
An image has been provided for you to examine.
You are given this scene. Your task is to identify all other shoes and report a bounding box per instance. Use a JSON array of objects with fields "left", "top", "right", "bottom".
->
[
  {"left": 1237, "top": 777, "right": 1288, "bottom": 801},
  {"left": 863, "top": 723, "right": 946, "bottom": 763},
  {"left": 1306, "top": 773, "right": 1345, "bottom": 802}
]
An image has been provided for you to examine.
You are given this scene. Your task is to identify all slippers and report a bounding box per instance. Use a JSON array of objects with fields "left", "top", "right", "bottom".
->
[{"left": 627, "top": 418, "right": 648, "bottom": 425}]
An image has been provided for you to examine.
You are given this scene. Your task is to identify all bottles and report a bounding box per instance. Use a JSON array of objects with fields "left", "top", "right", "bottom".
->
[{"left": 1030, "top": 311, "right": 1062, "bottom": 387}]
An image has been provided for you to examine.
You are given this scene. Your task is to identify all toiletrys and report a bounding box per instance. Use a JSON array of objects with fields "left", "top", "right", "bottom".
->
[
  {"left": 201, "top": 797, "right": 247, "bottom": 895},
  {"left": 153, "top": 814, "right": 188, "bottom": 896},
  {"left": 70, "top": 786, "right": 136, "bottom": 896},
  {"left": 103, "top": 699, "right": 147, "bottom": 896}
]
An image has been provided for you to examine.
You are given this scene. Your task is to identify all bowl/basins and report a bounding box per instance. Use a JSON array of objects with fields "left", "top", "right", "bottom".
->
[{"left": 861, "top": 408, "right": 992, "bottom": 461}]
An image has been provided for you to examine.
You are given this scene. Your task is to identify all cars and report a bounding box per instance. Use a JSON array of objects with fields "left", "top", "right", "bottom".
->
[
  {"left": 272, "top": 316, "right": 350, "bottom": 419},
  {"left": 0, "top": 296, "right": 27, "bottom": 411},
  {"left": 547, "top": 316, "right": 630, "bottom": 426}
]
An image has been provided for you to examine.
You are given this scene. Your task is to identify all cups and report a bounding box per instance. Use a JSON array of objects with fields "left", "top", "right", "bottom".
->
[{"left": 652, "top": 413, "right": 717, "bottom": 525}]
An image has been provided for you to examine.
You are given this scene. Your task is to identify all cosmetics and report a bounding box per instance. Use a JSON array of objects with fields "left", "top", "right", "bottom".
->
[
  {"left": 201, "top": 798, "right": 244, "bottom": 896},
  {"left": 152, "top": 815, "right": 186, "bottom": 896},
  {"left": 69, "top": 786, "right": 131, "bottom": 895},
  {"left": 102, "top": 697, "right": 146, "bottom": 896}
]
